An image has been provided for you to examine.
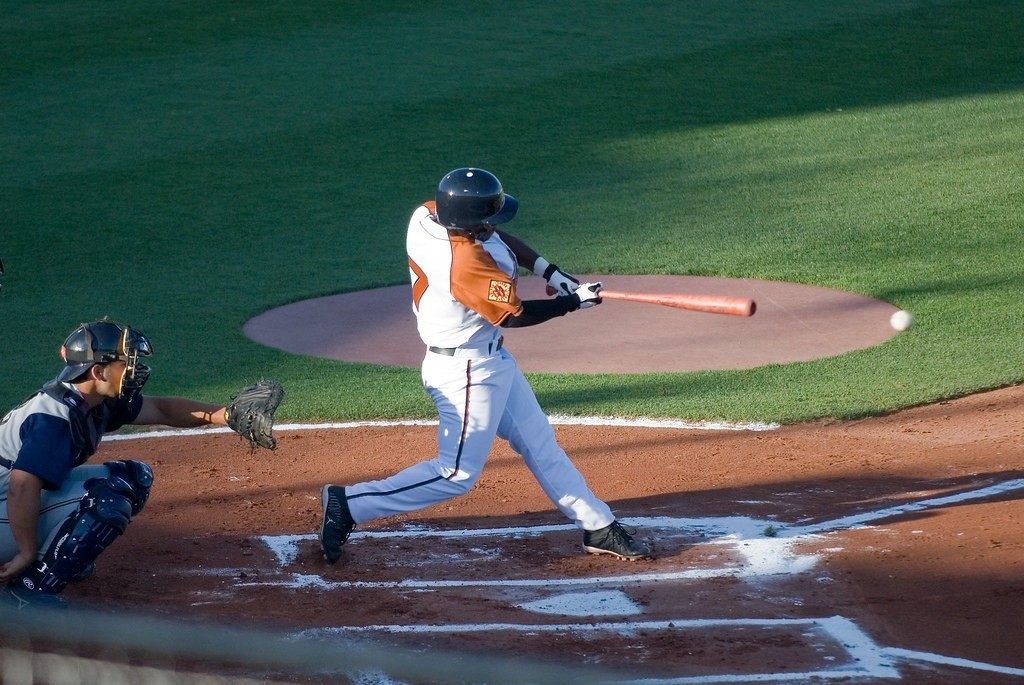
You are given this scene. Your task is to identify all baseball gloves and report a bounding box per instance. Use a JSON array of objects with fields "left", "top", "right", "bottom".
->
[{"left": 228, "top": 380, "right": 285, "bottom": 453}]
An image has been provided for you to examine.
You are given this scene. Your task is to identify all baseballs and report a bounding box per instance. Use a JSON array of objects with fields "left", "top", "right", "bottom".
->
[{"left": 890, "top": 309, "right": 913, "bottom": 333}]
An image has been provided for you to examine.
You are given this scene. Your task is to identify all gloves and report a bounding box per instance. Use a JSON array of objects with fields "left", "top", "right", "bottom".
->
[
  {"left": 543, "top": 262, "right": 579, "bottom": 296},
  {"left": 561, "top": 282, "right": 603, "bottom": 311}
]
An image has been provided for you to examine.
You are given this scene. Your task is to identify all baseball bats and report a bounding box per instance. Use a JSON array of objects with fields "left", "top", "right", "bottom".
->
[{"left": 544, "top": 283, "right": 758, "bottom": 318}]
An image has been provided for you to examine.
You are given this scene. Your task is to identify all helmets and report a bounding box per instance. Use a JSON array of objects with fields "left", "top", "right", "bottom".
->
[
  {"left": 57, "top": 319, "right": 129, "bottom": 383},
  {"left": 435, "top": 168, "right": 519, "bottom": 237}
]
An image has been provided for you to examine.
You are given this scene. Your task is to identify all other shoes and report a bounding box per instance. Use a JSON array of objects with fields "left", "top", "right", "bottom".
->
[{"left": 2, "top": 590, "right": 58, "bottom": 618}]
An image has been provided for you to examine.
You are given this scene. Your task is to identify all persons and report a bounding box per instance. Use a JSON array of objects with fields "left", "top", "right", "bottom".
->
[
  {"left": 317, "top": 167, "right": 650, "bottom": 566},
  {"left": 0, "top": 316, "right": 285, "bottom": 614}
]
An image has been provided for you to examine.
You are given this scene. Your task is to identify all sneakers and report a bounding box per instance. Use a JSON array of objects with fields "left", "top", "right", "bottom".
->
[
  {"left": 582, "top": 520, "right": 652, "bottom": 562},
  {"left": 321, "top": 483, "right": 358, "bottom": 567}
]
